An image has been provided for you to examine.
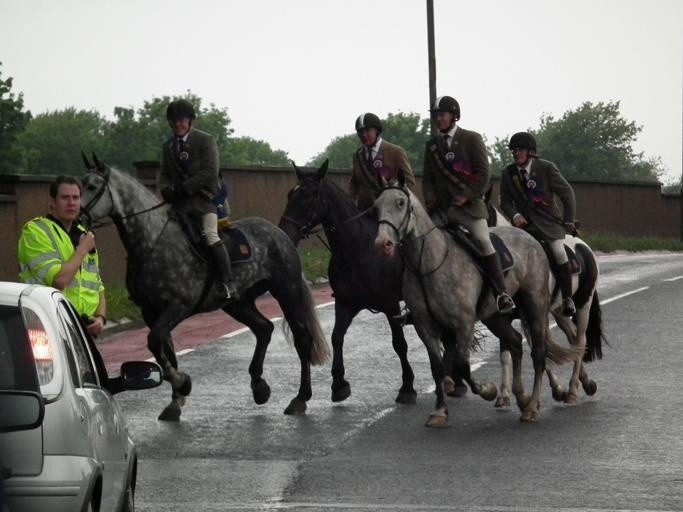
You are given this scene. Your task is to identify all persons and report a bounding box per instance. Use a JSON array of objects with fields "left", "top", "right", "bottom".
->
[
  {"left": 500, "top": 132, "right": 578, "bottom": 317},
  {"left": 393, "top": 95, "right": 512, "bottom": 323},
  {"left": 159, "top": 99, "right": 236, "bottom": 300},
  {"left": 17, "top": 174, "right": 107, "bottom": 343},
  {"left": 348, "top": 113, "right": 415, "bottom": 216}
]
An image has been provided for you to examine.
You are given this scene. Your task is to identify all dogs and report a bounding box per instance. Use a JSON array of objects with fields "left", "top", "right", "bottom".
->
[
  {"left": 277, "top": 158, "right": 491, "bottom": 405},
  {"left": 372, "top": 169, "right": 588, "bottom": 428},
  {"left": 481, "top": 183, "right": 612, "bottom": 410},
  {"left": 75, "top": 149, "right": 332, "bottom": 422}
]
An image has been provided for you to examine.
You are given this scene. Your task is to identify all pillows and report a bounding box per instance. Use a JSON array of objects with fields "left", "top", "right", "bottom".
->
[
  {"left": 510, "top": 132, "right": 536, "bottom": 150},
  {"left": 356, "top": 113, "right": 382, "bottom": 132},
  {"left": 167, "top": 101, "right": 195, "bottom": 119},
  {"left": 428, "top": 96, "right": 460, "bottom": 121}
]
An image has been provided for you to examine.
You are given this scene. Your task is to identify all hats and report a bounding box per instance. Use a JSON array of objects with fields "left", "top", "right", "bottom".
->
[{"left": 0, "top": 281, "right": 163, "bottom": 510}]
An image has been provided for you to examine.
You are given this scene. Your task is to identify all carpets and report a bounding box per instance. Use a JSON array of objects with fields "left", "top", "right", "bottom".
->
[
  {"left": 367, "top": 149, "right": 374, "bottom": 161},
  {"left": 443, "top": 135, "right": 449, "bottom": 151},
  {"left": 178, "top": 139, "right": 183, "bottom": 153},
  {"left": 520, "top": 169, "right": 529, "bottom": 188}
]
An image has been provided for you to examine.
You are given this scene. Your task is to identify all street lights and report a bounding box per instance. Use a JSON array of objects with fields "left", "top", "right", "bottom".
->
[
  {"left": 169, "top": 138, "right": 231, "bottom": 219},
  {"left": 428, "top": 138, "right": 497, "bottom": 227}
]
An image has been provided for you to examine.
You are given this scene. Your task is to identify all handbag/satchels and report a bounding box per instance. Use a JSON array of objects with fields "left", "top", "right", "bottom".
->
[{"left": 95, "top": 314, "right": 107, "bottom": 325}]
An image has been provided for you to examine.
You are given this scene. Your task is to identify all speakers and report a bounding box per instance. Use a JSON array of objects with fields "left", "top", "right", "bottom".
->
[
  {"left": 485, "top": 252, "right": 512, "bottom": 310},
  {"left": 210, "top": 241, "right": 237, "bottom": 297},
  {"left": 557, "top": 261, "right": 573, "bottom": 315}
]
